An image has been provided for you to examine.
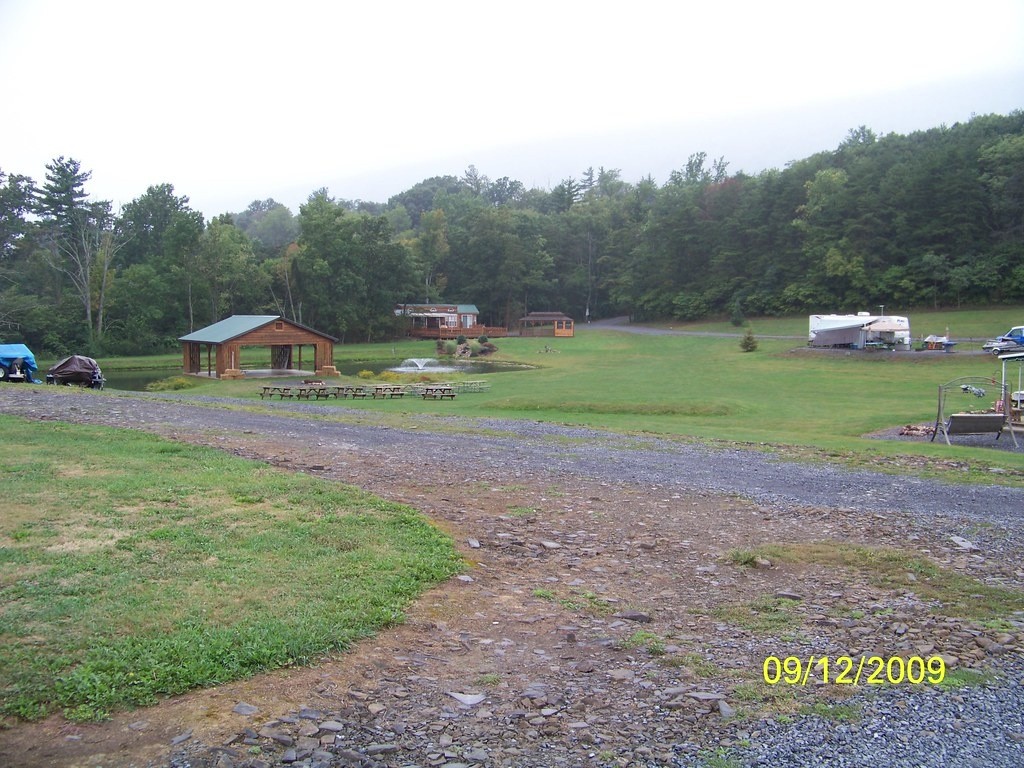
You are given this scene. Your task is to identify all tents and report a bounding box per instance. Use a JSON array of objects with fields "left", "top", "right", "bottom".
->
[{"left": 861, "top": 320, "right": 908, "bottom": 344}]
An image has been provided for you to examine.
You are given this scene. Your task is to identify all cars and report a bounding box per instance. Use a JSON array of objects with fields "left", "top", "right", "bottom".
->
[{"left": 981, "top": 326, "right": 1024, "bottom": 355}]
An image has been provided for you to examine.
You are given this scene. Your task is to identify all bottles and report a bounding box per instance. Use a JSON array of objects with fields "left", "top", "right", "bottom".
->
[
  {"left": 1020, "top": 400, "right": 1022, "bottom": 408},
  {"left": 990, "top": 400, "right": 1004, "bottom": 414}
]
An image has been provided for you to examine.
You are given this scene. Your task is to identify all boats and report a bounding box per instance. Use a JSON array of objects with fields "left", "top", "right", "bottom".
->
[{"left": 46, "top": 354, "right": 103, "bottom": 390}]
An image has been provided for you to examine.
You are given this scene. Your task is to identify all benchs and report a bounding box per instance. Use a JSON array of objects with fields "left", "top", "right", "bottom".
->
[
  {"left": 256, "top": 386, "right": 490, "bottom": 401},
  {"left": 942, "top": 341, "right": 958, "bottom": 353}
]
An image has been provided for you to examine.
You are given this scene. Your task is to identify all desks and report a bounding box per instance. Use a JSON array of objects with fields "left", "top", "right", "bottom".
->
[
  {"left": 430, "top": 382, "right": 457, "bottom": 394},
  {"left": 258, "top": 386, "right": 291, "bottom": 399},
  {"left": 460, "top": 380, "right": 486, "bottom": 392},
  {"left": 338, "top": 388, "right": 363, "bottom": 399},
  {"left": 376, "top": 387, "right": 402, "bottom": 399},
  {"left": 298, "top": 388, "right": 328, "bottom": 400},
  {"left": 426, "top": 388, "right": 453, "bottom": 398}
]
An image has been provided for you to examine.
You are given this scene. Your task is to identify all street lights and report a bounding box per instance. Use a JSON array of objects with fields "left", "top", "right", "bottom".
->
[{"left": 879, "top": 305, "right": 884, "bottom": 316}]
